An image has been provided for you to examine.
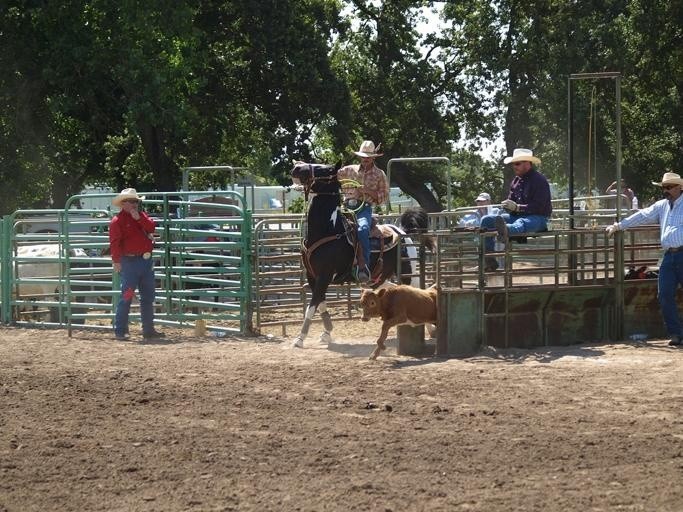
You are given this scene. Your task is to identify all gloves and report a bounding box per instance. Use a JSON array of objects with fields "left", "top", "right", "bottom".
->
[{"left": 606, "top": 221, "right": 621, "bottom": 239}]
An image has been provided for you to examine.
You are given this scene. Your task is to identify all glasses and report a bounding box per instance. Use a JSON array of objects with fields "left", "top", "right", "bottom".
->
[
  {"left": 659, "top": 184, "right": 681, "bottom": 190},
  {"left": 511, "top": 162, "right": 522, "bottom": 166},
  {"left": 123, "top": 198, "right": 140, "bottom": 204}
]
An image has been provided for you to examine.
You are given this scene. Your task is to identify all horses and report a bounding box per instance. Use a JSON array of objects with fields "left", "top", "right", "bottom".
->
[{"left": 291, "top": 158, "right": 436, "bottom": 348}]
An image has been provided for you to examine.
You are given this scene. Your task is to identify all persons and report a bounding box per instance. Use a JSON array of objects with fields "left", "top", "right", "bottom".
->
[
  {"left": 108, "top": 188, "right": 165, "bottom": 339},
  {"left": 477, "top": 148, "right": 551, "bottom": 272},
  {"left": 603, "top": 171, "right": 681, "bottom": 345},
  {"left": 605, "top": 177, "right": 632, "bottom": 207},
  {"left": 458, "top": 193, "right": 501, "bottom": 270},
  {"left": 335, "top": 140, "right": 389, "bottom": 280}
]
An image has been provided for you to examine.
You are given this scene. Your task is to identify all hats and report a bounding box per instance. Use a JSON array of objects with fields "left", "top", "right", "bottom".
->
[
  {"left": 476, "top": 193, "right": 491, "bottom": 202},
  {"left": 353, "top": 140, "right": 385, "bottom": 158},
  {"left": 652, "top": 172, "right": 683, "bottom": 190},
  {"left": 111, "top": 187, "right": 146, "bottom": 207},
  {"left": 503, "top": 148, "right": 542, "bottom": 164}
]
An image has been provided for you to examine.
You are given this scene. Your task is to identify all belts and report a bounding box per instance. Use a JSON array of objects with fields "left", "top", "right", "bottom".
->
[{"left": 667, "top": 246, "right": 683, "bottom": 252}]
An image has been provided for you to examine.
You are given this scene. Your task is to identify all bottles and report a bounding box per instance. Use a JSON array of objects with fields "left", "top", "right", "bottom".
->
[
  {"left": 487, "top": 205, "right": 493, "bottom": 216},
  {"left": 629, "top": 333, "right": 649, "bottom": 341},
  {"left": 631, "top": 197, "right": 638, "bottom": 209},
  {"left": 580, "top": 200, "right": 586, "bottom": 212}
]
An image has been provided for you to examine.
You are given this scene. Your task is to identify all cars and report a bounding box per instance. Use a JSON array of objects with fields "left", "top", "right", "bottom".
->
[{"left": 91, "top": 211, "right": 225, "bottom": 310}]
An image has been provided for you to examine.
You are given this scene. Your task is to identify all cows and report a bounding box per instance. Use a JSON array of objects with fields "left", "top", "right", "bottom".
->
[
  {"left": 360, "top": 285, "right": 438, "bottom": 361},
  {"left": 624, "top": 266, "right": 659, "bottom": 280},
  {"left": 12, "top": 205, "right": 232, "bottom": 324}
]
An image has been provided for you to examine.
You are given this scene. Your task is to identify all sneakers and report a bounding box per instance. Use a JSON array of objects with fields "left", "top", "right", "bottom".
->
[
  {"left": 115, "top": 330, "right": 164, "bottom": 341},
  {"left": 483, "top": 259, "right": 499, "bottom": 272},
  {"left": 495, "top": 216, "right": 507, "bottom": 242},
  {"left": 357, "top": 269, "right": 369, "bottom": 282},
  {"left": 668, "top": 333, "right": 683, "bottom": 346}
]
